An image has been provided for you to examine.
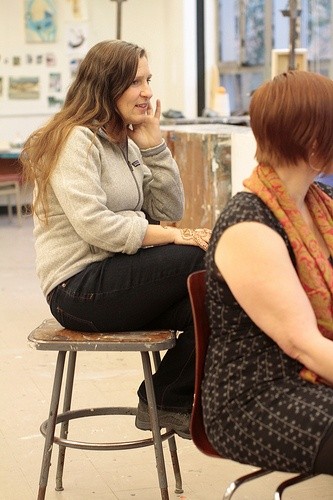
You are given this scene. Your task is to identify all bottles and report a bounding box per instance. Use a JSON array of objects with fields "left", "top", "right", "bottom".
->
[{"left": 213, "top": 86, "right": 230, "bottom": 117}]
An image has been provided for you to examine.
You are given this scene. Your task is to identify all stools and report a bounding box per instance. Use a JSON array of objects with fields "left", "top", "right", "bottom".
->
[{"left": 27, "top": 318, "right": 184, "bottom": 500}]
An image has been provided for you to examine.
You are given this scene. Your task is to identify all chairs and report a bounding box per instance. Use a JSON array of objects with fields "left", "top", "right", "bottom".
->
[{"left": 188, "top": 270, "right": 321, "bottom": 500}]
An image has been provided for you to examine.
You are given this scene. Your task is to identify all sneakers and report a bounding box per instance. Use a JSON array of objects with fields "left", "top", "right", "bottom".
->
[{"left": 135, "top": 396, "right": 193, "bottom": 440}]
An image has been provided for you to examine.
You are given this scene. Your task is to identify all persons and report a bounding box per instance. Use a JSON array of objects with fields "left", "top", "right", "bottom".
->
[
  {"left": 17, "top": 39, "right": 214, "bottom": 439},
  {"left": 201, "top": 69, "right": 333, "bottom": 476}
]
